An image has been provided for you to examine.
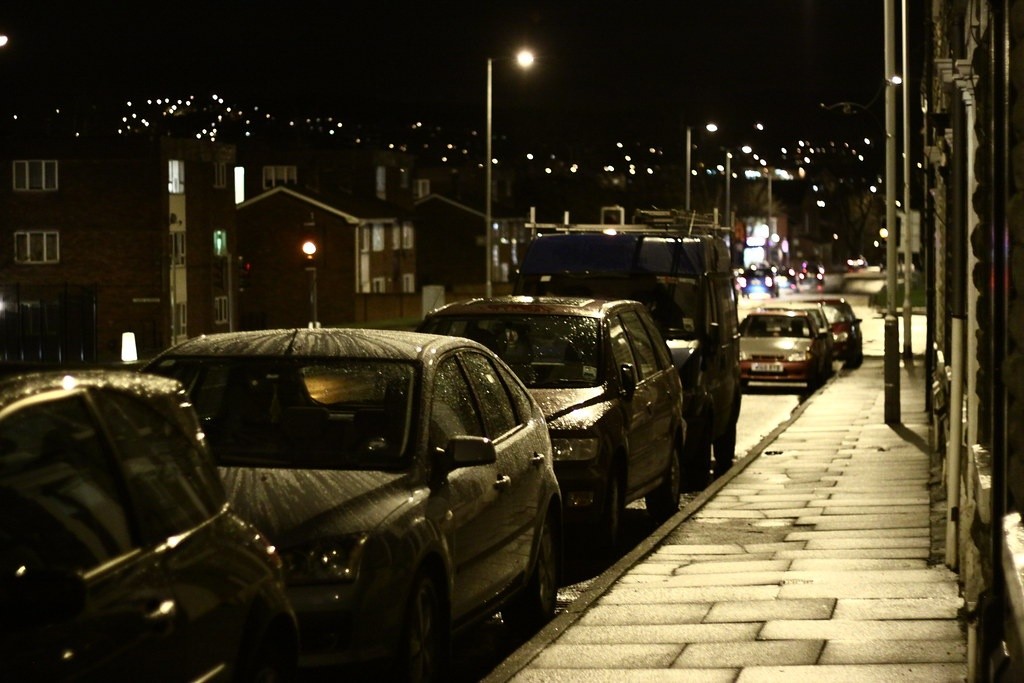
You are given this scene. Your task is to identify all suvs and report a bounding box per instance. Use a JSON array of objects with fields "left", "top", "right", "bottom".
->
[{"left": 417, "top": 294, "right": 687, "bottom": 563}]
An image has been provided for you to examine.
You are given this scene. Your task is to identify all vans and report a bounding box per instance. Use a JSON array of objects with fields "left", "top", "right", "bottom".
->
[{"left": 516, "top": 231, "right": 742, "bottom": 493}]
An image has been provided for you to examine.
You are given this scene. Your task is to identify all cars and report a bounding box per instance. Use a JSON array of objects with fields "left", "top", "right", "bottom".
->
[
  {"left": 740, "top": 255, "right": 866, "bottom": 370},
  {"left": 740, "top": 311, "right": 829, "bottom": 393},
  {"left": 136, "top": 325, "right": 564, "bottom": 683},
  {"left": 0, "top": 367, "right": 304, "bottom": 683}
]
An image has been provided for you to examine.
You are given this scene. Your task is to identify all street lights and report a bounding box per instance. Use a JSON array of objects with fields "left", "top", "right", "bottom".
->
[
  {"left": 724, "top": 146, "right": 752, "bottom": 225},
  {"left": 302, "top": 241, "right": 318, "bottom": 324},
  {"left": 483, "top": 48, "right": 535, "bottom": 299},
  {"left": 684, "top": 122, "right": 720, "bottom": 211}
]
